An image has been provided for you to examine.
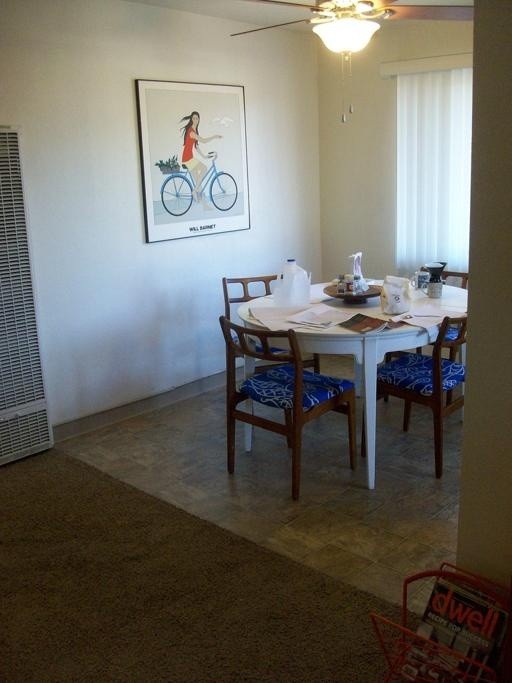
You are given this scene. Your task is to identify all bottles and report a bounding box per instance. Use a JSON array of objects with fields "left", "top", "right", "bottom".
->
[
  {"left": 336, "top": 274, "right": 344, "bottom": 293},
  {"left": 352, "top": 275, "right": 364, "bottom": 294}
]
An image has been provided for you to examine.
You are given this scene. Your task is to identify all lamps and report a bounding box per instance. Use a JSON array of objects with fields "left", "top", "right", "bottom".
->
[{"left": 311, "top": 18, "right": 380, "bottom": 123}]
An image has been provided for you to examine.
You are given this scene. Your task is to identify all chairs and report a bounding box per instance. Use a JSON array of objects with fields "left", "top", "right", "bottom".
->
[
  {"left": 361, "top": 316, "right": 467, "bottom": 479},
  {"left": 385, "top": 267, "right": 468, "bottom": 401},
  {"left": 219, "top": 316, "right": 356, "bottom": 502},
  {"left": 222, "top": 275, "right": 319, "bottom": 376}
]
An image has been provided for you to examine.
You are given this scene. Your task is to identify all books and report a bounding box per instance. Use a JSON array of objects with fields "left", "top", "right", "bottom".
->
[
  {"left": 334, "top": 313, "right": 406, "bottom": 334},
  {"left": 396, "top": 574, "right": 510, "bottom": 683}
]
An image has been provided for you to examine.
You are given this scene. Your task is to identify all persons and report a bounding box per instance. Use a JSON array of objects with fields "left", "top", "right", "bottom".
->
[{"left": 179, "top": 111, "right": 222, "bottom": 211}]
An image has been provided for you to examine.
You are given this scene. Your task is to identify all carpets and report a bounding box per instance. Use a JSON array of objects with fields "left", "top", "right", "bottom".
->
[{"left": 0, "top": 449, "right": 422, "bottom": 683}]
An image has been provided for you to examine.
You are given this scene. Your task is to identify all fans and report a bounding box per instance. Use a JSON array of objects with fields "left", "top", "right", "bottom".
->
[{"left": 229, "top": 0, "right": 474, "bottom": 37}]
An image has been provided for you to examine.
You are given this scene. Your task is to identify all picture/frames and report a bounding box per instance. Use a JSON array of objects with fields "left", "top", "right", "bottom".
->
[{"left": 135, "top": 79, "right": 251, "bottom": 243}]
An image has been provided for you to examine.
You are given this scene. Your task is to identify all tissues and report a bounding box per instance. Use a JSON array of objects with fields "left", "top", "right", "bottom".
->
[{"left": 380, "top": 276, "right": 414, "bottom": 316}]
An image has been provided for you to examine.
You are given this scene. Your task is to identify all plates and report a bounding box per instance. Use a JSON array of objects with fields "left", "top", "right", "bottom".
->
[{"left": 322, "top": 283, "right": 383, "bottom": 303}]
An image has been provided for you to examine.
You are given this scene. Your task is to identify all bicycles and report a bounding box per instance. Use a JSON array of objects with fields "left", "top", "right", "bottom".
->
[{"left": 161, "top": 151, "right": 237, "bottom": 216}]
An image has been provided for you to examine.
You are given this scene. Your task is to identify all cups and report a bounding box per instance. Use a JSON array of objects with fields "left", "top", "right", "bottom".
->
[
  {"left": 411, "top": 270, "right": 430, "bottom": 288},
  {"left": 421, "top": 260, "right": 447, "bottom": 298}
]
